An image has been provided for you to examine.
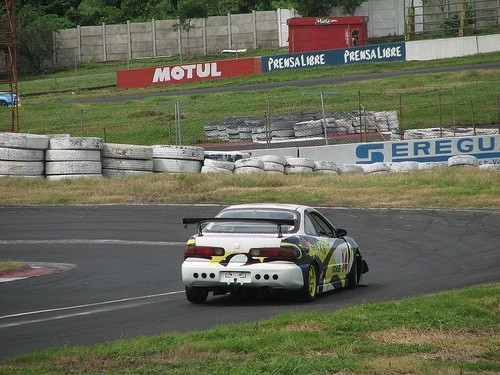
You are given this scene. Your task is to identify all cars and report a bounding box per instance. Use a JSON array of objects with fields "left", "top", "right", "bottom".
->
[
  {"left": 179, "top": 202, "right": 370, "bottom": 304},
  {"left": 0, "top": 93, "right": 20, "bottom": 106}
]
